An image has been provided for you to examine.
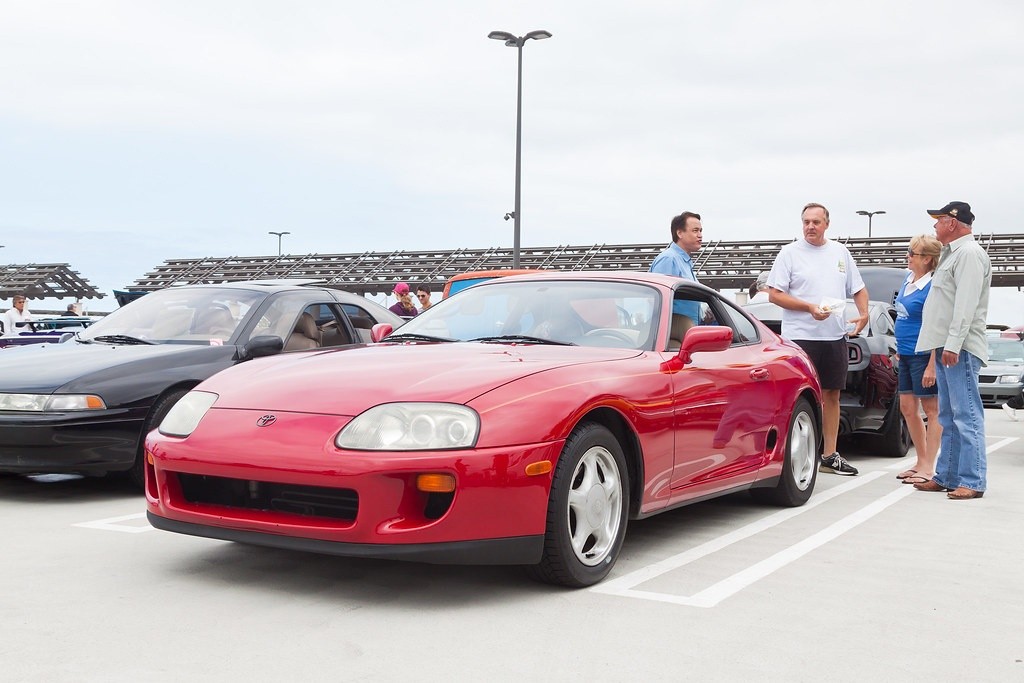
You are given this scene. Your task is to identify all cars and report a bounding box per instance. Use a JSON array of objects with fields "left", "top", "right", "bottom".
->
[
  {"left": 736, "top": 268, "right": 930, "bottom": 459},
  {"left": 977, "top": 324, "right": 1024, "bottom": 405},
  {"left": 441, "top": 268, "right": 622, "bottom": 332},
  {"left": 1, "top": 283, "right": 408, "bottom": 489}
]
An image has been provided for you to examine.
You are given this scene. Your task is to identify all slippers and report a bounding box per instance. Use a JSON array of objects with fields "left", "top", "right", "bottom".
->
[
  {"left": 902, "top": 476, "right": 932, "bottom": 484},
  {"left": 896, "top": 470, "right": 918, "bottom": 479}
]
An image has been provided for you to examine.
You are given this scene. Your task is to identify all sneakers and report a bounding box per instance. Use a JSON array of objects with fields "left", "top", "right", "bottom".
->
[{"left": 819, "top": 452, "right": 859, "bottom": 475}]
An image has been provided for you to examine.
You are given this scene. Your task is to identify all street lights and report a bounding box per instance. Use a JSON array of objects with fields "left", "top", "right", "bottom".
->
[
  {"left": 486, "top": 28, "right": 553, "bottom": 271},
  {"left": 267, "top": 231, "right": 291, "bottom": 276},
  {"left": 856, "top": 211, "right": 887, "bottom": 264}
]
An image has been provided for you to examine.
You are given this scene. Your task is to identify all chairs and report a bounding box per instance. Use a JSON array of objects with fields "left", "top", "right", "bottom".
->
[
  {"left": 531, "top": 317, "right": 582, "bottom": 340},
  {"left": 276, "top": 311, "right": 318, "bottom": 351},
  {"left": 638, "top": 316, "right": 695, "bottom": 352},
  {"left": 188, "top": 304, "right": 233, "bottom": 336}
]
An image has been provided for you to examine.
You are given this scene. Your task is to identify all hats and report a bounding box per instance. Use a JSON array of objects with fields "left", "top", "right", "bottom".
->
[
  {"left": 926, "top": 201, "right": 975, "bottom": 226},
  {"left": 392, "top": 282, "right": 409, "bottom": 294}
]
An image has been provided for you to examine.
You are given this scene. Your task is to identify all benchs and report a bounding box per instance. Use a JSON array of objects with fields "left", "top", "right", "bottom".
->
[{"left": 259, "top": 316, "right": 376, "bottom": 347}]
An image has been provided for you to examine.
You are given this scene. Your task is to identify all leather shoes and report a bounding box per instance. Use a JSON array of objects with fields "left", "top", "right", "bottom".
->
[
  {"left": 947, "top": 486, "right": 984, "bottom": 499},
  {"left": 913, "top": 478, "right": 954, "bottom": 492}
]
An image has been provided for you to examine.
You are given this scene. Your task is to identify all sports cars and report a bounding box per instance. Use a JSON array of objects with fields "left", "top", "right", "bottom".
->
[{"left": 142, "top": 269, "right": 826, "bottom": 590}]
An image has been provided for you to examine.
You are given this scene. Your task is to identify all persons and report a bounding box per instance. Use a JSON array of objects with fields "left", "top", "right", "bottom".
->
[
  {"left": 894, "top": 233, "right": 943, "bottom": 484},
  {"left": 913, "top": 201, "right": 992, "bottom": 500},
  {"left": 647, "top": 212, "right": 703, "bottom": 327},
  {"left": 766, "top": 203, "right": 869, "bottom": 475},
  {"left": 2, "top": 295, "right": 31, "bottom": 338},
  {"left": 749, "top": 271, "right": 770, "bottom": 306},
  {"left": 388, "top": 282, "right": 417, "bottom": 322},
  {"left": 417, "top": 284, "right": 435, "bottom": 315},
  {"left": 61, "top": 303, "right": 79, "bottom": 316}
]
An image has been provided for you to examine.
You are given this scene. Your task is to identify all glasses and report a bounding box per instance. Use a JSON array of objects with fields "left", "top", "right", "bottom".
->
[
  {"left": 19, "top": 302, "right": 24, "bottom": 304},
  {"left": 417, "top": 295, "right": 425, "bottom": 298},
  {"left": 907, "top": 247, "right": 928, "bottom": 258}
]
